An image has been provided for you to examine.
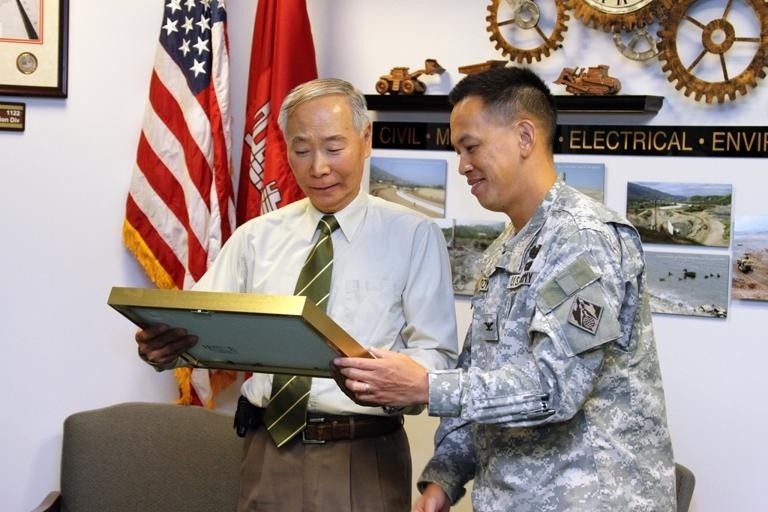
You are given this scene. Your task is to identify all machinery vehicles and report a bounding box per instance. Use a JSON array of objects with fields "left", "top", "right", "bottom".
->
[
  {"left": 470, "top": 231, "right": 493, "bottom": 250},
  {"left": 736, "top": 251, "right": 754, "bottom": 275}
]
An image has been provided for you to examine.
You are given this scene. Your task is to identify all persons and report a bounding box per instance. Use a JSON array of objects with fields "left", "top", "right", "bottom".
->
[
  {"left": 135, "top": 77, "right": 459, "bottom": 512},
  {"left": 334, "top": 66, "right": 681, "bottom": 512}
]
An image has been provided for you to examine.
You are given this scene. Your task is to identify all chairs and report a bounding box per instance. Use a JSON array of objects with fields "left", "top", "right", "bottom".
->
[
  {"left": 32, "top": 401, "right": 243, "bottom": 512},
  {"left": 675, "top": 462, "right": 695, "bottom": 512}
]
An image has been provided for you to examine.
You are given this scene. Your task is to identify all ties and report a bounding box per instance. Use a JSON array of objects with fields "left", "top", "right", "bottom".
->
[{"left": 263, "top": 215, "right": 339, "bottom": 450}]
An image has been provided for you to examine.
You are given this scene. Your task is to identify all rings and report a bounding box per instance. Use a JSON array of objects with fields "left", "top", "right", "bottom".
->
[{"left": 363, "top": 382, "right": 371, "bottom": 393}]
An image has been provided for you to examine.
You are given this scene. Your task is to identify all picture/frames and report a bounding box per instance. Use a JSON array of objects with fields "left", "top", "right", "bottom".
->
[{"left": 0, "top": 0, "right": 70, "bottom": 98}]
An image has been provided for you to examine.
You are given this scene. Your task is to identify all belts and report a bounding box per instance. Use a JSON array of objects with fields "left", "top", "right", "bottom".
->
[{"left": 301, "top": 417, "right": 401, "bottom": 444}]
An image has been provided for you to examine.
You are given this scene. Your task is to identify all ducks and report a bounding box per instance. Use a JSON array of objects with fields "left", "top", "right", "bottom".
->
[{"left": 682, "top": 269, "right": 696, "bottom": 279}]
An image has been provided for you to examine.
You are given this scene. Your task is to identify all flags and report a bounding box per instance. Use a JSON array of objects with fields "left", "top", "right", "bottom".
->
[
  {"left": 122, "top": 0, "right": 237, "bottom": 408},
  {"left": 237, "top": 1, "right": 321, "bottom": 382}
]
